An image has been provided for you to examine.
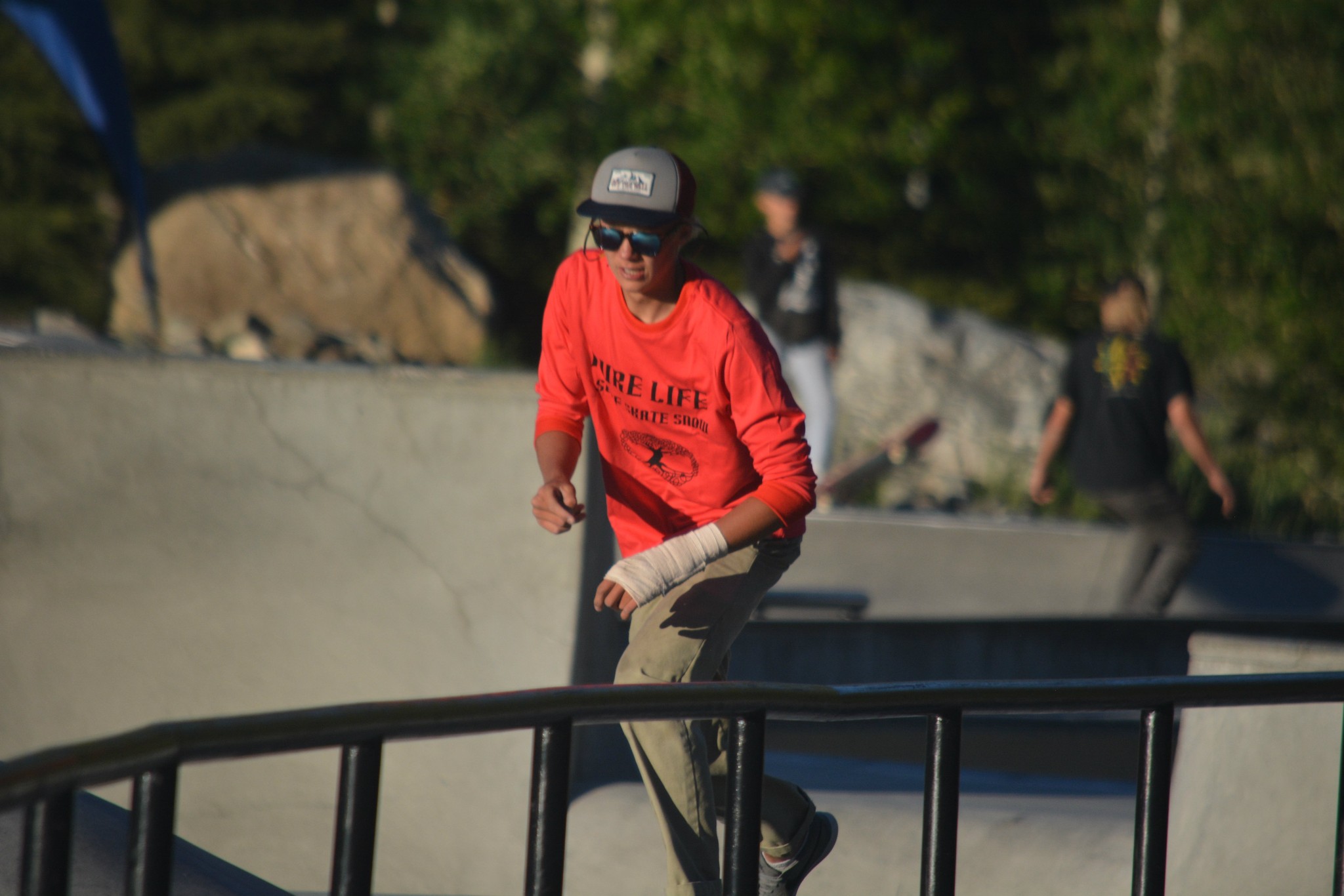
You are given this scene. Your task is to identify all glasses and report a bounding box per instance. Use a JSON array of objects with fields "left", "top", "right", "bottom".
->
[{"left": 588, "top": 217, "right": 676, "bottom": 256}]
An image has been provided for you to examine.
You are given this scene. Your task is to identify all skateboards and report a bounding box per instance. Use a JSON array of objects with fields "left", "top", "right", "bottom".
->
[{"left": 814, "top": 418, "right": 941, "bottom": 512}]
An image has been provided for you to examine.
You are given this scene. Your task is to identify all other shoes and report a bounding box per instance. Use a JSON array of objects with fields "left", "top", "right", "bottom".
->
[{"left": 756, "top": 810, "right": 839, "bottom": 896}]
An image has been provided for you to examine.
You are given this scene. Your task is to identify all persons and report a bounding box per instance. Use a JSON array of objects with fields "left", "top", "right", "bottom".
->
[
  {"left": 728, "top": 165, "right": 845, "bottom": 514},
  {"left": 530, "top": 144, "right": 841, "bottom": 896},
  {"left": 1029, "top": 270, "right": 1235, "bottom": 618}
]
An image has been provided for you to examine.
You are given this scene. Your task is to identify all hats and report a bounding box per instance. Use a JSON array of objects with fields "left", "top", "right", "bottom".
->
[{"left": 576, "top": 147, "right": 696, "bottom": 227}]
